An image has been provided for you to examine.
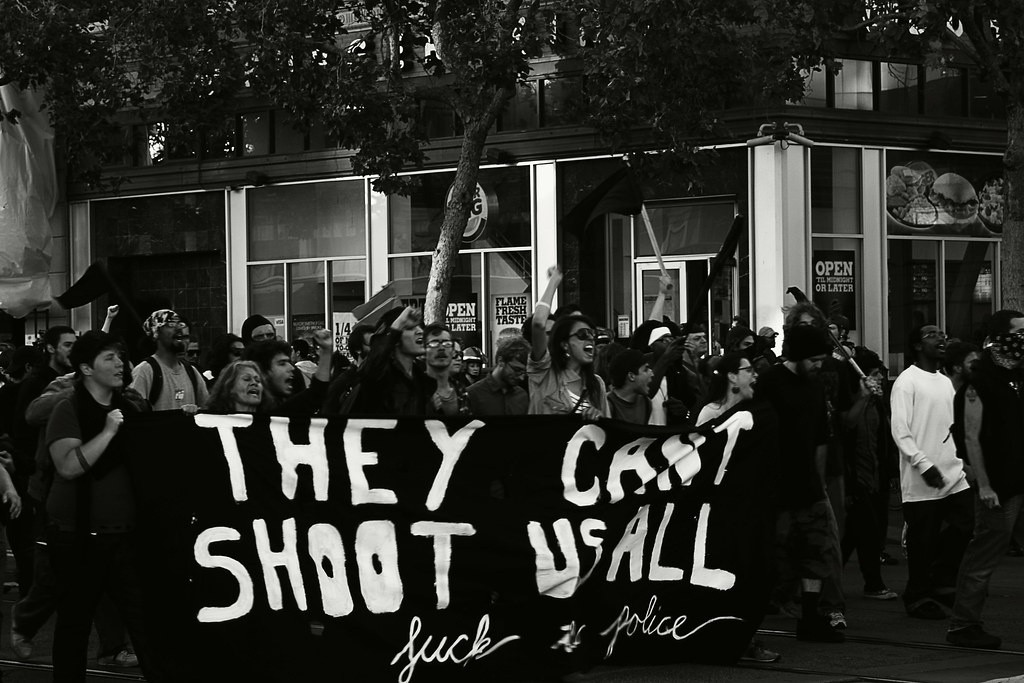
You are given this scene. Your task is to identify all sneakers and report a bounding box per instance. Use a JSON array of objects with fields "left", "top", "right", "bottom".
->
[{"left": 741, "top": 644, "right": 782, "bottom": 663}]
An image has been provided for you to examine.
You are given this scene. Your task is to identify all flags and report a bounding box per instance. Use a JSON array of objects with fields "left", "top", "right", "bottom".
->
[
  {"left": 560, "top": 166, "right": 644, "bottom": 251},
  {"left": 53, "top": 261, "right": 112, "bottom": 310}
]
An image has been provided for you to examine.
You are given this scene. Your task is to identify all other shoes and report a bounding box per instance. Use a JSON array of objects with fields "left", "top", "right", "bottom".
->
[
  {"left": 907, "top": 601, "right": 946, "bottom": 620},
  {"left": 878, "top": 552, "right": 899, "bottom": 566},
  {"left": 863, "top": 580, "right": 899, "bottom": 600},
  {"left": 778, "top": 601, "right": 800, "bottom": 619},
  {"left": 3, "top": 581, "right": 19, "bottom": 593},
  {"left": 938, "top": 592, "right": 957, "bottom": 610},
  {"left": 1004, "top": 546, "right": 1024, "bottom": 558},
  {"left": 795, "top": 618, "right": 845, "bottom": 643},
  {"left": 8, "top": 604, "right": 36, "bottom": 659},
  {"left": 828, "top": 610, "right": 849, "bottom": 630},
  {"left": 943, "top": 620, "right": 1002, "bottom": 652},
  {"left": 95, "top": 646, "right": 139, "bottom": 668}
]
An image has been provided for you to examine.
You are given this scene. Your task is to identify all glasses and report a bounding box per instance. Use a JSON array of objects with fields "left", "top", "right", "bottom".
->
[
  {"left": 450, "top": 349, "right": 465, "bottom": 360},
  {"left": 567, "top": 327, "right": 597, "bottom": 342},
  {"left": 919, "top": 331, "right": 947, "bottom": 343},
  {"left": 658, "top": 335, "right": 673, "bottom": 345},
  {"left": 423, "top": 339, "right": 454, "bottom": 350},
  {"left": 162, "top": 322, "right": 185, "bottom": 328}
]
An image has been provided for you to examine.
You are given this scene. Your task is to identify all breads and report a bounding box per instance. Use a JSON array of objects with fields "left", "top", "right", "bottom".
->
[{"left": 886, "top": 161, "right": 937, "bottom": 199}]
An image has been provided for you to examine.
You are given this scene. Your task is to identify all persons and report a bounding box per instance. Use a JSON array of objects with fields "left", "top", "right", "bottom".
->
[
  {"left": 125, "top": 269, "right": 713, "bottom": 427},
  {"left": 0, "top": 304, "right": 152, "bottom": 683},
  {"left": 695, "top": 301, "right": 1024, "bottom": 663}
]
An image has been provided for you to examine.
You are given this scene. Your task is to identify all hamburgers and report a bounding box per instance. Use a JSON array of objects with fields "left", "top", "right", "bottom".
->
[{"left": 928, "top": 172, "right": 980, "bottom": 225}]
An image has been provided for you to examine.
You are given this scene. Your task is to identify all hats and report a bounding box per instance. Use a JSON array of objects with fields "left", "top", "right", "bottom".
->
[
  {"left": 141, "top": 308, "right": 178, "bottom": 341},
  {"left": 724, "top": 326, "right": 758, "bottom": 355},
  {"left": 758, "top": 326, "right": 779, "bottom": 339},
  {"left": 629, "top": 319, "right": 671, "bottom": 346},
  {"left": 463, "top": 346, "right": 487, "bottom": 364},
  {"left": 67, "top": 328, "right": 122, "bottom": 370}
]
